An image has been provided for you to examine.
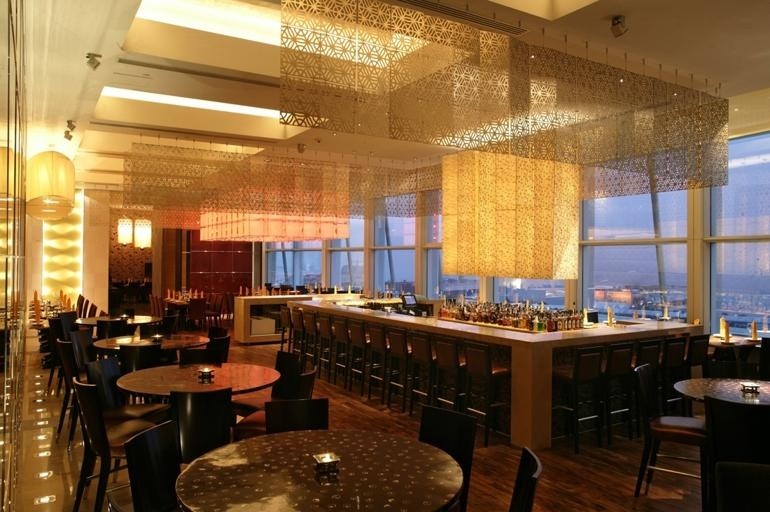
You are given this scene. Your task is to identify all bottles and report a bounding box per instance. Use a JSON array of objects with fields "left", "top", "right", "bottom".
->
[{"left": 438, "top": 302, "right": 585, "bottom": 333}]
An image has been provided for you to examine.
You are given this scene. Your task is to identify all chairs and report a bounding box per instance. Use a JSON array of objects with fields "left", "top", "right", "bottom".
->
[
  {"left": 28, "top": 281, "right": 318, "bottom": 487},
  {"left": 125, "top": 420, "right": 186, "bottom": 512},
  {"left": 510, "top": 446, "right": 541, "bottom": 511},
  {"left": 72, "top": 378, "right": 156, "bottom": 511},
  {"left": 265, "top": 398, "right": 328, "bottom": 434},
  {"left": 551, "top": 333, "right": 770, "bottom": 512},
  {"left": 420, "top": 407, "right": 477, "bottom": 512}
]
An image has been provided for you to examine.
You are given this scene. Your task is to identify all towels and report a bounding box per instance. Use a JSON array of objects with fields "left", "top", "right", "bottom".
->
[{"left": 583, "top": 300, "right": 769, "bottom": 342}]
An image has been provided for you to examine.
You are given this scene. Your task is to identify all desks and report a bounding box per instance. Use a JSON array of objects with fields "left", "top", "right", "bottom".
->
[{"left": 176, "top": 429, "right": 462, "bottom": 512}]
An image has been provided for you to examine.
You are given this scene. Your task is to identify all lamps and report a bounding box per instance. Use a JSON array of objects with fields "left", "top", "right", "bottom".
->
[
  {"left": 196, "top": 188, "right": 349, "bottom": 244},
  {"left": 440, "top": 150, "right": 581, "bottom": 283},
  {"left": 115, "top": 210, "right": 153, "bottom": 253},
  {"left": 26, "top": 136, "right": 78, "bottom": 221}
]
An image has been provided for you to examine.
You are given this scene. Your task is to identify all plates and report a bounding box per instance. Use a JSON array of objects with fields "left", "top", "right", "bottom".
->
[
  {"left": 720, "top": 340, "right": 735, "bottom": 344},
  {"left": 745, "top": 336, "right": 762, "bottom": 341},
  {"left": 713, "top": 333, "right": 732, "bottom": 338}
]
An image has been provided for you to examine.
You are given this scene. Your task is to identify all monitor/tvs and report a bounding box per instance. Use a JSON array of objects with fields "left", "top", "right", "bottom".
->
[{"left": 402, "top": 295, "right": 418, "bottom": 306}]
